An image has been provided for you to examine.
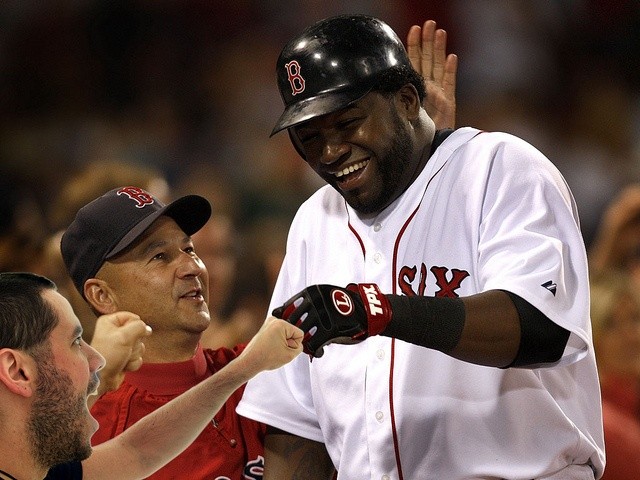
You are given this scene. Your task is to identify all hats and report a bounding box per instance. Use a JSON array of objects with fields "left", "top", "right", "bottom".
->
[{"left": 61, "top": 185, "right": 212, "bottom": 296}]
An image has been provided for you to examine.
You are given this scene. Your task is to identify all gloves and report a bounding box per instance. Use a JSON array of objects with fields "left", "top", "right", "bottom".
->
[{"left": 271, "top": 283, "right": 393, "bottom": 363}]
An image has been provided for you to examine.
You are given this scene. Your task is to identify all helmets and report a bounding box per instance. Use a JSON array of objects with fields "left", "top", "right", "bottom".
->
[{"left": 269, "top": 14, "right": 414, "bottom": 138}]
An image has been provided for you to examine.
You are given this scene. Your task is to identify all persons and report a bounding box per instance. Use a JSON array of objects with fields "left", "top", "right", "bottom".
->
[
  {"left": 58, "top": 18, "right": 457, "bottom": 480},
  {"left": 0, "top": 271, "right": 304, "bottom": 480},
  {"left": 86, "top": 310, "right": 152, "bottom": 408},
  {"left": 235, "top": 15, "right": 607, "bottom": 480}
]
